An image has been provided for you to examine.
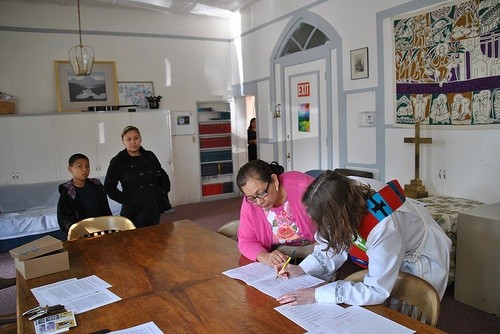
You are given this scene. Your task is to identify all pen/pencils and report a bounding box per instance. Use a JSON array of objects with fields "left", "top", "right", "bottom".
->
[{"left": 276, "top": 250, "right": 295, "bottom": 279}]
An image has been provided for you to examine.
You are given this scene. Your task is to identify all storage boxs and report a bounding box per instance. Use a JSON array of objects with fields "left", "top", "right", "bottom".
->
[{"left": 9, "top": 234, "right": 70, "bottom": 280}]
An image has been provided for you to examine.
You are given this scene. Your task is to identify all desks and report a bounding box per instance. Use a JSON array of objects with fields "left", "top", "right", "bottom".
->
[{"left": 16, "top": 219, "right": 450, "bottom": 334}]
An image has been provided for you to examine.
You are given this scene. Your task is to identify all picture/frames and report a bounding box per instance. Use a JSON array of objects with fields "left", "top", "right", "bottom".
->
[
  {"left": 170, "top": 110, "right": 197, "bottom": 135},
  {"left": 53, "top": 61, "right": 155, "bottom": 113},
  {"left": 349, "top": 47, "right": 369, "bottom": 81}
]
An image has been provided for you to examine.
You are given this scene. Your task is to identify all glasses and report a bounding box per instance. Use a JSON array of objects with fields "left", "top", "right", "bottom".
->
[{"left": 244, "top": 176, "right": 272, "bottom": 203}]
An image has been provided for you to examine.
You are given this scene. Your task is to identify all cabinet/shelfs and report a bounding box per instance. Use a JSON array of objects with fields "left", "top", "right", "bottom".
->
[
  {"left": 1, "top": 109, "right": 176, "bottom": 211},
  {"left": 384, "top": 129, "right": 500, "bottom": 205}
]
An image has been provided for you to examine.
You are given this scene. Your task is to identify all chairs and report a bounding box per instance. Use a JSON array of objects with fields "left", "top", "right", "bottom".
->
[
  {"left": 343, "top": 269, "right": 440, "bottom": 327},
  {"left": 67, "top": 215, "right": 136, "bottom": 241}
]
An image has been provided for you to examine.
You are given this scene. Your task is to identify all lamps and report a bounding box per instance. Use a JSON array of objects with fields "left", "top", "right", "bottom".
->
[{"left": 68, "top": 0, "right": 95, "bottom": 76}]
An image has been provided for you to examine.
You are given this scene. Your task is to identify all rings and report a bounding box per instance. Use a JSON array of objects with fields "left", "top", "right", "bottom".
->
[{"left": 294, "top": 296, "right": 296, "bottom": 301}]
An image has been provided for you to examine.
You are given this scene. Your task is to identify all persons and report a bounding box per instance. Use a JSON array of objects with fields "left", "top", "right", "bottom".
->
[
  {"left": 104, "top": 126, "right": 173, "bottom": 228},
  {"left": 57, "top": 153, "right": 113, "bottom": 237},
  {"left": 275, "top": 171, "right": 453, "bottom": 324},
  {"left": 247, "top": 118, "right": 257, "bottom": 162},
  {"left": 236, "top": 159, "right": 318, "bottom": 267}
]
